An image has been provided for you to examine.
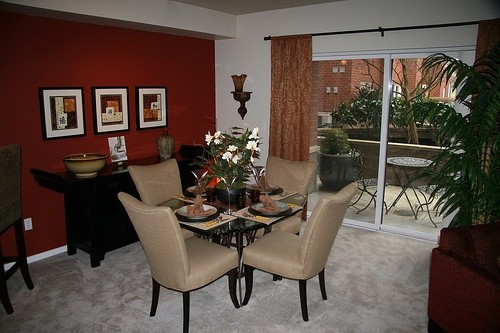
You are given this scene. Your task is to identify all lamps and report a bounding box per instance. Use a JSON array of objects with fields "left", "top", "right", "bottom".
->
[{"left": 231, "top": 74, "right": 252, "bottom": 120}]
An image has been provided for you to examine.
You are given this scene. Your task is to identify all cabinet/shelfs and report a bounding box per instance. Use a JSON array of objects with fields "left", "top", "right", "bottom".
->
[{"left": 29, "top": 144, "right": 214, "bottom": 267}]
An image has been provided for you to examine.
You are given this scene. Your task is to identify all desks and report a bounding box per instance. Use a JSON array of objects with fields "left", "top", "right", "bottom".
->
[
  {"left": 386, "top": 157, "right": 436, "bottom": 218},
  {"left": 156, "top": 186, "right": 307, "bottom": 279}
]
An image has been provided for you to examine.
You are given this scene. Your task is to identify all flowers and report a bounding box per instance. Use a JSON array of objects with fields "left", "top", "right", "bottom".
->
[{"left": 190, "top": 126, "right": 263, "bottom": 188}]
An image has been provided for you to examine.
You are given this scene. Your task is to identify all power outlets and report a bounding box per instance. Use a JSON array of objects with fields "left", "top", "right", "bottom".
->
[{"left": 24, "top": 218, "right": 33, "bottom": 230}]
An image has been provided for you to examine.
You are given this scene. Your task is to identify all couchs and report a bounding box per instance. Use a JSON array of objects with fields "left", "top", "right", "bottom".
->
[{"left": 427, "top": 224, "right": 500, "bottom": 333}]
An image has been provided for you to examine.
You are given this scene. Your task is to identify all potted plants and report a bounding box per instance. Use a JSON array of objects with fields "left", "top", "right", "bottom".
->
[{"left": 316, "top": 127, "right": 360, "bottom": 188}]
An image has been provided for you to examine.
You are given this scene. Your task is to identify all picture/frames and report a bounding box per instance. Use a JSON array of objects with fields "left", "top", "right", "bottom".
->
[
  {"left": 40, "top": 86, "right": 86, "bottom": 140},
  {"left": 92, "top": 86, "right": 130, "bottom": 135},
  {"left": 135, "top": 86, "right": 168, "bottom": 130}
]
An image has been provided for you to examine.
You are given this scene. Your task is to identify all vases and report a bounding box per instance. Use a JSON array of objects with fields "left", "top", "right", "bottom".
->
[{"left": 214, "top": 184, "right": 246, "bottom": 205}]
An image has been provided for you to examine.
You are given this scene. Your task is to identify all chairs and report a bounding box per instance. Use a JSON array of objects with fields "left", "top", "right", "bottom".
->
[
  {"left": 415, "top": 181, "right": 449, "bottom": 227},
  {"left": 118, "top": 191, "right": 240, "bottom": 333},
  {"left": 0, "top": 144, "right": 34, "bottom": 314},
  {"left": 127, "top": 158, "right": 194, "bottom": 241},
  {"left": 348, "top": 148, "right": 388, "bottom": 215},
  {"left": 243, "top": 179, "right": 361, "bottom": 321},
  {"left": 250, "top": 156, "right": 317, "bottom": 281}
]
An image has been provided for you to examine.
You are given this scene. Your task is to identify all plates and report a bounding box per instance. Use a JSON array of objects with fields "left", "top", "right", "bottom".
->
[
  {"left": 177, "top": 204, "right": 217, "bottom": 220},
  {"left": 186, "top": 186, "right": 206, "bottom": 196},
  {"left": 247, "top": 184, "right": 279, "bottom": 194},
  {"left": 251, "top": 201, "right": 289, "bottom": 215}
]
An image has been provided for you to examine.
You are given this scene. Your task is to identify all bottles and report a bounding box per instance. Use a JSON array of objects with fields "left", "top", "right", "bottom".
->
[{"left": 155, "top": 129, "right": 175, "bottom": 159}]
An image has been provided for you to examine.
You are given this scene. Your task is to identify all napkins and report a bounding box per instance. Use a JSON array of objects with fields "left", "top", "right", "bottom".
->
[
  {"left": 187, "top": 194, "right": 213, "bottom": 216},
  {"left": 259, "top": 193, "right": 284, "bottom": 212},
  {"left": 191, "top": 180, "right": 207, "bottom": 193},
  {"left": 250, "top": 175, "right": 275, "bottom": 192}
]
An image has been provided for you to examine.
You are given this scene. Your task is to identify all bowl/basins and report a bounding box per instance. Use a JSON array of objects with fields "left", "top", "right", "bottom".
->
[{"left": 60, "top": 153, "right": 110, "bottom": 177}]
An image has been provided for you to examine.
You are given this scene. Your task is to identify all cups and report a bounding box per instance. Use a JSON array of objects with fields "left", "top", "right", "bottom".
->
[
  {"left": 250, "top": 189, "right": 261, "bottom": 203},
  {"left": 205, "top": 188, "right": 216, "bottom": 202},
  {"left": 235, "top": 195, "right": 246, "bottom": 209}
]
